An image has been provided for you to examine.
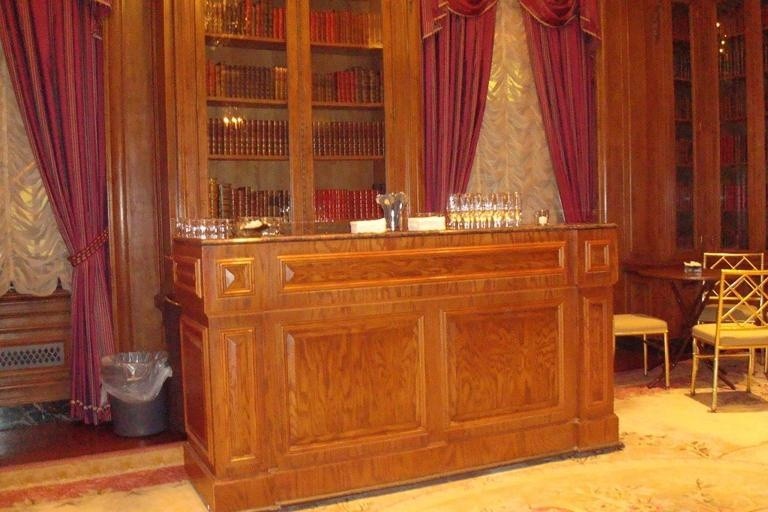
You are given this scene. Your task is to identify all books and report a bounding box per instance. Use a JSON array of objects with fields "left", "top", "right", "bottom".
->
[
  {"left": 206, "top": 116, "right": 384, "bottom": 158},
  {"left": 206, "top": 177, "right": 385, "bottom": 222},
  {"left": 205, "top": 58, "right": 382, "bottom": 104},
  {"left": 201, "top": 0, "right": 381, "bottom": 45}
]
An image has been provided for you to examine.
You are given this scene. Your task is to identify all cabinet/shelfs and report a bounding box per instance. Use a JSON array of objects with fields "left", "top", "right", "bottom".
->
[
  {"left": 153, "top": 0, "right": 425, "bottom": 225},
  {"left": 647, "top": 0, "right": 768, "bottom": 340}
]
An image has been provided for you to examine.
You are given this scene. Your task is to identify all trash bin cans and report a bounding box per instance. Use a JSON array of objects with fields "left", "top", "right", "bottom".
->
[{"left": 100, "top": 351, "right": 170, "bottom": 437}]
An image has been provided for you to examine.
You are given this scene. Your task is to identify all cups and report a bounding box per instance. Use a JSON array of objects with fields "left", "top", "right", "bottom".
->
[
  {"left": 446, "top": 192, "right": 524, "bottom": 230},
  {"left": 535, "top": 209, "right": 550, "bottom": 226},
  {"left": 174, "top": 216, "right": 235, "bottom": 239}
]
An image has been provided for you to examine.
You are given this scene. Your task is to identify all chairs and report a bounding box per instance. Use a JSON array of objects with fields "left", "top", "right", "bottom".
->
[
  {"left": 691, "top": 269, "right": 768, "bottom": 413},
  {"left": 612, "top": 313, "right": 670, "bottom": 387},
  {"left": 695, "top": 252, "right": 768, "bottom": 375}
]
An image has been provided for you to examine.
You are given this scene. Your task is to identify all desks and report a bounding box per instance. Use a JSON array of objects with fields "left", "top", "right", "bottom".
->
[{"left": 637, "top": 268, "right": 736, "bottom": 390}]
